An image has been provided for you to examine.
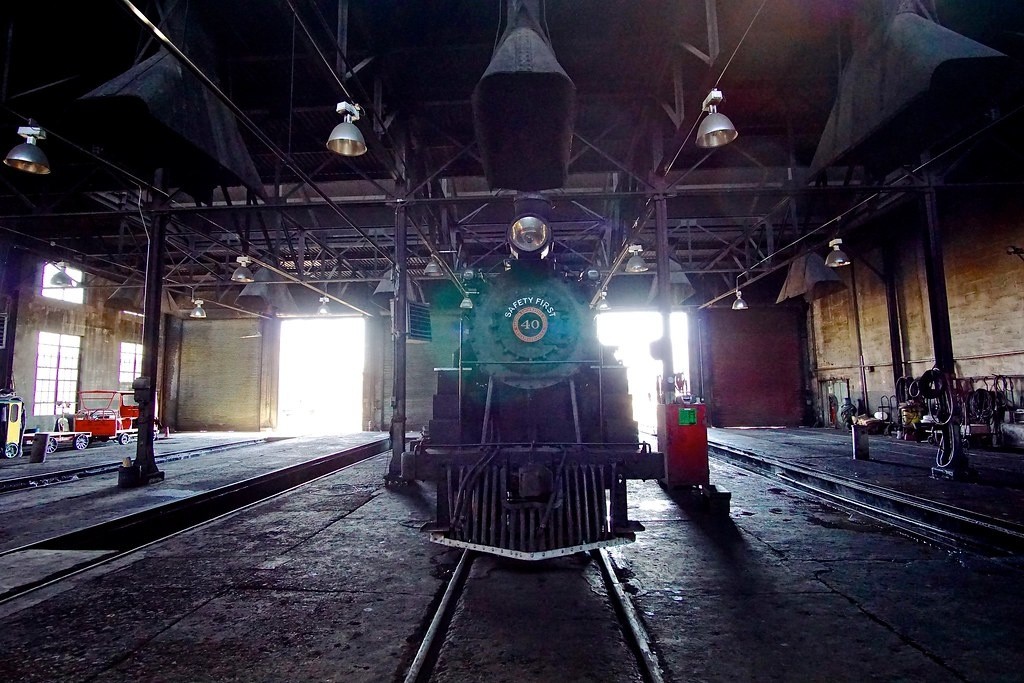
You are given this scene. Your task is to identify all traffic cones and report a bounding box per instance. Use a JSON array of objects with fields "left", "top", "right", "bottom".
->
[{"left": 164, "top": 426, "right": 170, "bottom": 439}]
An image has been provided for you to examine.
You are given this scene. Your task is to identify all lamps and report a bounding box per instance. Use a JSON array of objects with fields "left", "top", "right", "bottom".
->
[
  {"left": 231, "top": 256, "right": 255, "bottom": 283},
  {"left": 823, "top": 215, "right": 850, "bottom": 268},
  {"left": 731, "top": 290, "right": 748, "bottom": 310},
  {"left": 189, "top": 299, "right": 207, "bottom": 319},
  {"left": 423, "top": 254, "right": 443, "bottom": 276},
  {"left": 3, "top": 118, "right": 51, "bottom": 174},
  {"left": 693, "top": 86, "right": 738, "bottom": 149},
  {"left": 49, "top": 262, "right": 73, "bottom": 287},
  {"left": 625, "top": 244, "right": 648, "bottom": 273},
  {"left": 326, "top": 102, "right": 367, "bottom": 157}
]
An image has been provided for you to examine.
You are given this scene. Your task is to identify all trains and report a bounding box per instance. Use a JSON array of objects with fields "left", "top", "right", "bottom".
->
[{"left": 398, "top": 254, "right": 668, "bottom": 562}]
